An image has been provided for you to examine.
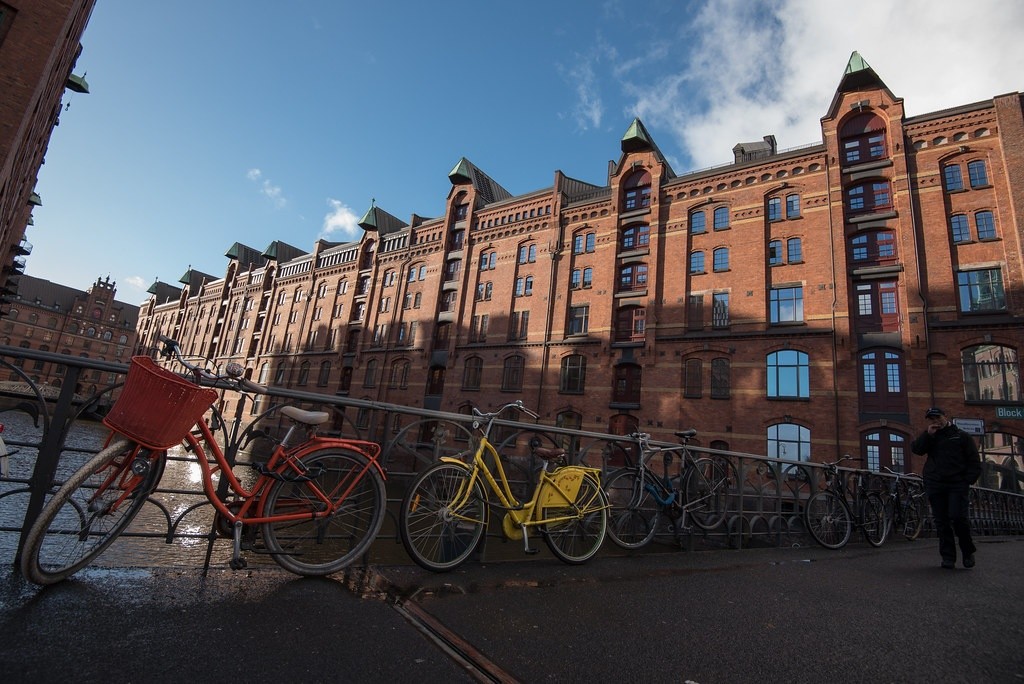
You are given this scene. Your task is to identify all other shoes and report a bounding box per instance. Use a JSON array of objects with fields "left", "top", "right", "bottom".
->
[
  {"left": 941, "top": 558, "right": 955, "bottom": 568},
  {"left": 963, "top": 554, "right": 975, "bottom": 567}
]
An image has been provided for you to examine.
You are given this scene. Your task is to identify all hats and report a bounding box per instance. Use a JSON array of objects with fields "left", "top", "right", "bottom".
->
[{"left": 925, "top": 407, "right": 944, "bottom": 418}]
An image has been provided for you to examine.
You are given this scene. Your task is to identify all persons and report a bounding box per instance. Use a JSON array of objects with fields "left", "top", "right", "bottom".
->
[{"left": 912, "top": 407, "right": 981, "bottom": 568}]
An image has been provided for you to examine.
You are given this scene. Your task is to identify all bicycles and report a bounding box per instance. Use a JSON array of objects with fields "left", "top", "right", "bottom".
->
[
  {"left": 804, "top": 453, "right": 889, "bottom": 549},
  {"left": 873, "top": 465, "right": 928, "bottom": 540},
  {"left": 19, "top": 334, "right": 388, "bottom": 589},
  {"left": 397, "top": 395, "right": 612, "bottom": 572},
  {"left": 598, "top": 424, "right": 731, "bottom": 550}
]
